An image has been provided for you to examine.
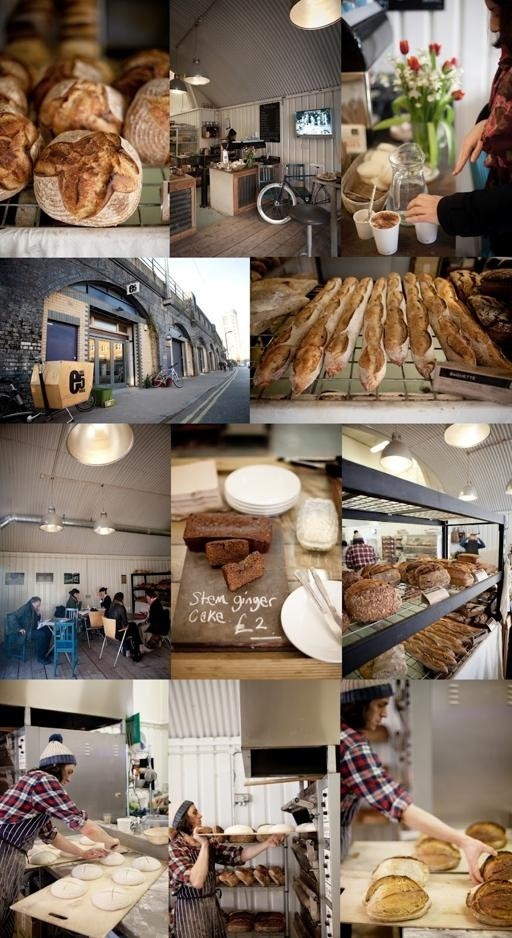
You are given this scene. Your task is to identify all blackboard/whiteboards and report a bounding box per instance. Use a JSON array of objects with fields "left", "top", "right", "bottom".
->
[{"left": 260, "top": 102, "right": 280, "bottom": 143}]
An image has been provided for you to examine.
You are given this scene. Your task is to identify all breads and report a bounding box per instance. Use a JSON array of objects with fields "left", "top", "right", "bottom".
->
[
  {"left": 342, "top": 553, "right": 497, "bottom": 680},
  {"left": 183, "top": 511, "right": 273, "bottom": 593},
  {"left": 227, "top": 910, "right": 285, "bottom": 933},
  {"left": 0, "top": 0, "right": 171, "bottom": 228},
  {"left": 363, "top": 821, "right": 512, "bottom": 927},
  {"left": 356, "top": 143, "right": 397, "bottom": 192},
  {"left": 219, "top": 864, "right": 285, "bottom": 888},
  {"left": 250, "top": 268, "right": 512, "bottom": 396}
]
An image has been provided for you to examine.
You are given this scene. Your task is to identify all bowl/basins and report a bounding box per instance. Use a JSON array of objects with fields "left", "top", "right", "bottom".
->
[{"left": 143, "top": 826, "right": 168, "bottom": 846}]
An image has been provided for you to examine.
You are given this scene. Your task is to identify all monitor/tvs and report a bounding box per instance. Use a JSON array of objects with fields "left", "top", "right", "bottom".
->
[{"left": 295, "top": 108, "right": 333, "bottom": 138}]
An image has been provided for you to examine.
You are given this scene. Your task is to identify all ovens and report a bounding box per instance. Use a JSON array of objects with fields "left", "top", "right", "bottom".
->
[{"left": 282, "top": 772, "right": 340, "bottom": 937}]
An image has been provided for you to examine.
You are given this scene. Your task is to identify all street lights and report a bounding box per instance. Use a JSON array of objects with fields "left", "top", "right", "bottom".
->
[{"left": 224, "top": 330, "right": 234, "bottom": 360}]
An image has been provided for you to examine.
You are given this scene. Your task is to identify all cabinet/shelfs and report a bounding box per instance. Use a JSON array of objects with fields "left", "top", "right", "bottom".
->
[
  {"left": 168, "top": 827, "right": 289, "bottom": 937},
  {"left": 168, "top": 129, "right": 198, "bottom": 154},
  {"left": 178, "top": 154, "right": 221, "bottom": 187},
  {"left": 341, "top": 455, "right": 505, "bottom": 679},
  {"left": 131, "top": 570, "right": 171, "bottom": 618}
]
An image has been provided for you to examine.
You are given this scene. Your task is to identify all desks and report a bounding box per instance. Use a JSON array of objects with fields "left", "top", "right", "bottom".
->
[
  {"left": 45, "top": 850, "right": 169, "bottom": 938},
  {"left": 314, "top": 173, "right": 341, "bottom": 256}
]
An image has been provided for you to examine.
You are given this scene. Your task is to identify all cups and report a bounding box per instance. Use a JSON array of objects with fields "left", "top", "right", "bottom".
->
[
  {"left": 117, "top": 817, "right": 131, "bottom": 832},
  {"left": 352, "top": 207, "right": 440, "bottom": 256},
  {"left": 102, "top": 811, "right": 113, "bottom": 826}
]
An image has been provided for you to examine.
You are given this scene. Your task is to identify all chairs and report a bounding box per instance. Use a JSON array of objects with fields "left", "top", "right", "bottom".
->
[
  {"left": 4, "top": 600, "right": 171, "bottom": 677},
  {"left": 257, "top": 164, "right": 282, "bottom": 221},
  {"left": 285, "top": 162, "right": 314, "bottom": 206}
]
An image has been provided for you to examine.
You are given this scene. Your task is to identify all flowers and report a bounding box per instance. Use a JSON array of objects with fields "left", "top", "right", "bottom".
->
[
  {"left": 373, "top": 30, "right": 465, "bottom": 168},
  {"left": 244, "top": 145, "right": 256, "bottom": 168}
]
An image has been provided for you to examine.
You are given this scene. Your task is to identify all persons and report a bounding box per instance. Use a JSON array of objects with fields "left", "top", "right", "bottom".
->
[
  {"left": 402, "top": 1, "right": 510, "bottom": 258},
  {"left": 341, "top": 531, "right": 376, "bottom": 574},
  {"left": 168, "top": 800, "right": 285, "bottom": 938},
  {"left": 218, "top": 360, "right": 233, "bottom": 372},
  {"left": 340, "top": 679, "right": 498, "bottom": 886},
  {"left": 225, "top": 129, "right": 237, "bottom": 152},
  {"left": 458, "top": 533, "right": 486, "bottom": 554},
  {"left": 2, "top": 732, "right": 120, "bottom": 937},
  {"left": 7, "top": 595, "right": 52, "bottom": 666},
  {"left": 106, "top": 591, "right": 156, "bottom": 655},
  {"left": 98, "top": 588, "right": 111, "bottom": 616},
  {"left": 394, "top": 546, "right": 407, "bottom": 566},
  {"left": 137, "top": 587, "right": 170, "bottom": 643},
  {"left": 66, "top": 588, "right": 82, "bottom": 613}
]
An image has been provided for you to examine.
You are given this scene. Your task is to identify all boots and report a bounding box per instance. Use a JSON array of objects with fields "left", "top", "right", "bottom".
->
[{"left": 139, "top": 644, "right": 154, "bottom": 654}]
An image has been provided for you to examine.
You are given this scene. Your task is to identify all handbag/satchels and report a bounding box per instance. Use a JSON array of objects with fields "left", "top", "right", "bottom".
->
[{"left": 54, "top": 605, "right": 66, "bottom": 618}]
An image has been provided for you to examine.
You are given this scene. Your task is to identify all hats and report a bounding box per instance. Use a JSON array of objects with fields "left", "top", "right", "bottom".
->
[
  {"left": 340, "top": 679, "right": 395, "bottom": 704},
  {"left": 228, "top": 128, "right": 237, "bottom": 137},
  {"left": 352, "top": 530, "right": 364, "bottom": 544},
  {"left": 38, "top": 733, "right": 76, "bottom": 768},
  {"left": 168, "top": 799, "right": 194, "bottom": 830}
]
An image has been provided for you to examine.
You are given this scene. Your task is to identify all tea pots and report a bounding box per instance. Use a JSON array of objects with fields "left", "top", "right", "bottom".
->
[{"left": 387, "top": 141, "right": 428, "bottom": 226}]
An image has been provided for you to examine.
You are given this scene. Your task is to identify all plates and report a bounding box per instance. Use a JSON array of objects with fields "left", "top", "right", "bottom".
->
[
  {"left": 171, "top": 455, "right": 303, "bottom": 527},
  {"left": 279, "top": 580, "right": 343, "bottom": 664},
  {"left": 294, "top": 495, "right": 340, "bottom": 552}
]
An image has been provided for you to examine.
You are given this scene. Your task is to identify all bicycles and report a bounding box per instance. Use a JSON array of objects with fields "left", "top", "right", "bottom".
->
[
  {"left": 255, "top": 162, "right": 338, "bottom": 225},
  {"left": 147, "top": 361, "right": 185, "bottom": 389}
]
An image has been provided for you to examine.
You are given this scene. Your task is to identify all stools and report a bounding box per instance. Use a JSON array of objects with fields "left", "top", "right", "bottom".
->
[{"left": 288, "top": 203, "right": 330, "bottom": 257}]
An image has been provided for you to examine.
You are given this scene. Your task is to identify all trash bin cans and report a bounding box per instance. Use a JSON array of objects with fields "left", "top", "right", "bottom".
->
[{"left": 93, "top": 387, "right": 113, "bottom": 408}]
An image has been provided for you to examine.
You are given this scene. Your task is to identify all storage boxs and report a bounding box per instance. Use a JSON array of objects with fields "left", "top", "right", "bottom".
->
[{"left": 15, "top": 886, "right": 41, "bottom": 937}]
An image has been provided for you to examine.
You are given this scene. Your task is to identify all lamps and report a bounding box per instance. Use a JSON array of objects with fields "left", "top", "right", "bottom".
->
[
  {"left": 442, "top": 423, "right": 493, "bottom": 449},
  {"left": 38, "top": 476, "right": 66, "bottom": 532},
  {"left": 92, "top": 482, "right": 117, "bottom": 533},
  {"left": 115, "top": 305, "right": 123, "bottom": 311},
  {"left": 458, "top": 451, "right": 479, "bottom": 501},
  {"left": 287, "top": 0, "right": 342, "bottom": 32},
  {"left": 63, "top": 421, "right": 136, "bottom": 465},
  {"left": 182, "top": 16, "right": 211, "bottom": 86},
  {"left": 379, "top": 423, "right": 413, "bottom": 472}
]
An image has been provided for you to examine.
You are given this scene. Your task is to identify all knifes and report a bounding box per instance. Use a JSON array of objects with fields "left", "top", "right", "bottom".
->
[{"left": 292, "top": 564, "right": 341, "bottom": 647}]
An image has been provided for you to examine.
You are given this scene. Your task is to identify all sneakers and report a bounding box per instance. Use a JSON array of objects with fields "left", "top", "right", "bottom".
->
[{"left": 37, "top": 655, "right": 51, "bottom": 666}]
